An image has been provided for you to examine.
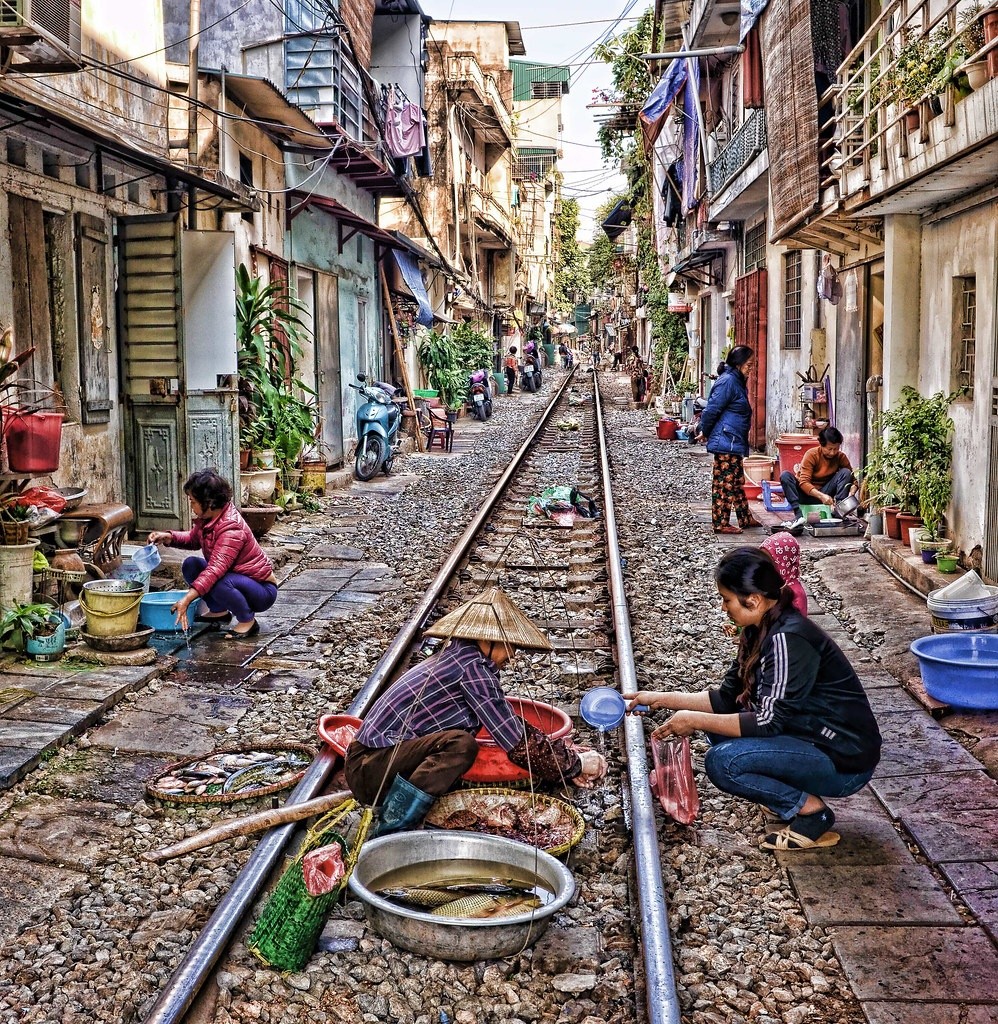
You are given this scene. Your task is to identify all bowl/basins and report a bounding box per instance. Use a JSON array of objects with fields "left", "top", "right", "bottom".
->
[
  {"left": 743, "top": 481, "right": 780, "bottom": 500},
  {"left": 579, "top": 687, "right": 648, "bottom": 729},
  {"left": 911, "top": 632, "right": 998, "bottom": 708},
  {"left": 351, "top": 833, "right": 575, "bottom": 961},
  {"left": 132, "top": 542, "right": 161, "bottom": 572},
  {"left": 771, "top": 526, "right": 804, "bottom": 535},
  {"left": 424, "top": 397, "right": 440, "bottom": 409},
  {"left": 461, "top": 697, "right": 572, "bottom": 781},
  {"left": 414, "top": 389, "right": 439, "bottom": 398},
  {"left": 139, "top": 592, "right": 202, "bottom": 632}
]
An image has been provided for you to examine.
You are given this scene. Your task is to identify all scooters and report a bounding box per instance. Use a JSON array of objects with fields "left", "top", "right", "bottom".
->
[
  {"left": 349, "top": 374, "right": 403, "bottom": 481},
  {"left": 464, "top": 368, "right": 492, "bottom": 422},
  {"left": 522, "top": 342, "right": 543, "bottom": 392}
]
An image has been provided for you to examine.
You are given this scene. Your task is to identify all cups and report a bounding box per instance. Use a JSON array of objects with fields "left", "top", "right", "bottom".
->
[{"left": 807, "top": 512, "right": 820, "bottom": 523}]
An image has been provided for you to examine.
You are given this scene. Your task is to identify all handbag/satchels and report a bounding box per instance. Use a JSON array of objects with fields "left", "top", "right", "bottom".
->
[
  {"left": 648, "top": 729, "right": 701, "bottom": 825},
  {"left": 248, "top": 796, "right": 373, "bottom": 972}
]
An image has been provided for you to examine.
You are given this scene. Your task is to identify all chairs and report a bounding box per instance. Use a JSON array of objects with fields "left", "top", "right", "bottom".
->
[{"left": 426, "top": 407, "right": 455, "bottom": 454}]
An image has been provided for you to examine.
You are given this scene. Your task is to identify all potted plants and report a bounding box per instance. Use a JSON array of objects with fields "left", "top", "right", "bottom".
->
[
  {"left": 953, "top": 43, "right": 973, "bottom": 95},
  {"left": 417, "top": 331, "right": 471, "bottom": 423},
  {"left": 850, "top": 384, "right": 970, "bottom": 546},
  {"left": 0, "top": 501, "right": 34, "bottom": 545},
  {"left": 669, "top": 392, "right": 683, "bottom": 415},
  {"left": 0, "top": 597, "right": 66, "bottom": 663},
  {"left": 240, "top": 414, "right": 273, "bottom": 470},
  {"left": 935, "top": 547, "right": 959, "bottom": 574},
  {"left": 957, "top": 0, "right": 987, "bottom": 91},
  {"left": 660, "top": 399, "right": 674, "bottom": 413},
  {"left": 914, "top": 468, "right": 952, "bottom": 565},
  {"left": 251, "top": 435, "right": 281, "bottom": 470},
  {"left": 929, "top": 77, "right": 945, "bottom": 112}
]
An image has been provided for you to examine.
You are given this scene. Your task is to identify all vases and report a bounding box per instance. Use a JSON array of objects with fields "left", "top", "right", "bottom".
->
[
  {"left": 240, "top": 471, "right": 256, "bottom": 506},
  {"left": 241, "top": 503, "right": 284, "bottom": 539},
  {"left": 241, "top": 466, "right": 282, "bottom": 499},
  {"left": 905, "top": 107, "right": 933, "bottom": 134},
  {"left": 907, "top": 527, "right": 938, "bottom": 555},
  {"left": 282, "top": 467, "right": 304, "bottom": 493},
  {"left": 980, "top": 7, "right": 998, "bottom": 79}
]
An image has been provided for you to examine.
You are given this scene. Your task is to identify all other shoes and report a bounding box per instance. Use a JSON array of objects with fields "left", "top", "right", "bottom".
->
[
  {"left": 738, "top": 521, "right": 763, "bottom": 528},
  {"left": 224, "top": 618, "right": 260, "bottom": 641},
  {"left": 712, "top": 523, "right": 744, "bottom": 535},
  {"left": 193, "top": 610, "right": 233, "bottom": 625}
]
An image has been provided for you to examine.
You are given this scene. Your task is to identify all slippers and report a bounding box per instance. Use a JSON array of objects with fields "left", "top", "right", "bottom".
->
[{"left": 759, "top": 823, "right": 841, "bottom": 851}]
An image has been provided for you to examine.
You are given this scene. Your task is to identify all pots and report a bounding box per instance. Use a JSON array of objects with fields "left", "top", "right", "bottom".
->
[{"left": 834, "top": 494, "right": 860, "bottom": 517}]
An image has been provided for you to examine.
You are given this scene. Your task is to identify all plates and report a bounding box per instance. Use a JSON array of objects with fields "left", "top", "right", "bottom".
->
[{"left": 820, "top": 519, "right": 842, "bottom": 523}]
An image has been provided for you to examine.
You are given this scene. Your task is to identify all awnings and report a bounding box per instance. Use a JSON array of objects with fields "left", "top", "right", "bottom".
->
[
  {"left": 198, "top": 69, "right": 336, "bottom": 150},
  {"left": 286, "top": 189, "right": 410, "bottom": 263},
  {"left": 601, "top": 200, "right": 630, "bottom": 243},
  {"left": 663, "top": 248, "right": 724, "bottom": 288},
  {"left": 433, "top": 312, "right": 459, "bottom": 334},
  {"left": 378, "top": 230, "right": 472, "bottom": 314}
]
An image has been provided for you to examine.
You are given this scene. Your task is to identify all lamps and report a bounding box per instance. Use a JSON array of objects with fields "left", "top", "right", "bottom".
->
[{"left": 719, "top": 11, "right": 740, "bottom": 26}]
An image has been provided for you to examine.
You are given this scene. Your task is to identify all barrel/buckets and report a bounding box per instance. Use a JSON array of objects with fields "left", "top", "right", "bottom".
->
[
  {"left": 79, "top": 589, "right": 145, "bottom": 636},
  {"left": 2, "top": 405, "right": 64, "bottom": 472},
  {"left": 105, "top": 544, "right": 151, "bottom": 594},
  {"left": 657, "top": 421, "right": 678, "bottom": 438},
  {"left": 928, "top": 585, "right": 998, "bottom": 635},
  {"left": 743, "top": 461, "right": 772, "bottom": 483}
]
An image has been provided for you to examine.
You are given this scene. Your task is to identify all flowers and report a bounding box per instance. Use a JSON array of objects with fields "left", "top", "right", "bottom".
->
[{"left": 893, "top": 21, "right": 954, "bottom": 101}]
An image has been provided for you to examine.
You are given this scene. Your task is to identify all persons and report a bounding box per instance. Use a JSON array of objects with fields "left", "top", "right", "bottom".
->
[
  {"left": 694, "top": 345, "right": 763, "bottom": 534},
  {"left": 779, "top": 427, "right": 858, "bottom": 525},
  {"left": 626, "top": 346, "right": 644, "bottom": 401},
  {"left": 591, "top": 337, "right": 601, "bottom": 371},
  {"left": 146, "top": 471, "right": 278, "bottom": 640},
  {"left": 612, "top": 352, "right": 621, "bottom": 368},
  {"left": 559, "top": 343, "right": 573, "bottom": 370},
  {"left": 344, "top": 586, "right": 608, "bottom": 841},
  {"left": 721, "top": 532, "right": 808, "bottom": 637},
  {"left": 620, "top": 546, "right": 882, "bottom": 851},
  {"left": 507, "top": 346, "right": 517, "bottom": 397}
]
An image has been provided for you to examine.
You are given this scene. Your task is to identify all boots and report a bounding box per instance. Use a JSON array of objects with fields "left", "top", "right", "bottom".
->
[{"left": 368, "top": 773, "right": 436, "bottom": 843}]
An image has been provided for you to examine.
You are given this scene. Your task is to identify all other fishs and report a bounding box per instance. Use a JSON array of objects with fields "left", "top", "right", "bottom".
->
[{"left": 376, "top": 879, "right": 549, "bottom": 919}]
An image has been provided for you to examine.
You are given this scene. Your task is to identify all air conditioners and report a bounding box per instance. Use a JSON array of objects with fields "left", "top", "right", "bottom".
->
[{"left": 0, "top": 0, "right": 81, "bottom": 64}]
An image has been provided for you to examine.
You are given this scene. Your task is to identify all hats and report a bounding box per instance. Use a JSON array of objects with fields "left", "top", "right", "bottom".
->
[{"left": 421, "top": 586, "right": 556, "bottom": 651}]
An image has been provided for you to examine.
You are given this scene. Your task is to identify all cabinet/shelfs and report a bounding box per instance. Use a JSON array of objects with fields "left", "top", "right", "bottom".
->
[{"left": 795, "top": 391, "right": 829, "bottom": 430}]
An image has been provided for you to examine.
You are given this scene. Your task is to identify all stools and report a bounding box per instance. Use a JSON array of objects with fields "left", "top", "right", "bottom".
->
[
  {"left": 761, "top": 479, "right": 793, "bottom": 512},
  {"left": 799, "top": 505, "right": 832, "bottom": 519}
]
